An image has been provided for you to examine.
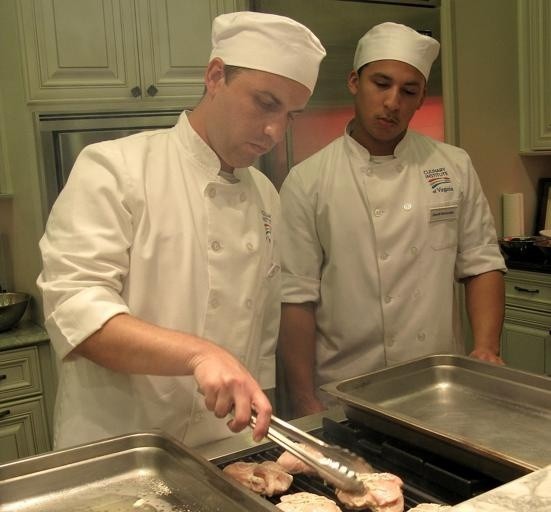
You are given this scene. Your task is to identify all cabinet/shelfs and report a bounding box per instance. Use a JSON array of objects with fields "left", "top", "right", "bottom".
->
[
  {"left": 459, "top": 1, "right": 551, "bottom": 378},
  {"left": 0, "top": 0, "right": 249, "bottom": 115},
  {"left": 1, "top": 322, "right": 52, "bottom": 466}
]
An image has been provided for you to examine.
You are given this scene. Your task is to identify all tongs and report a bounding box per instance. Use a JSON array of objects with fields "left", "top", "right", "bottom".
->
[{"left": 196, "top": 384, "right": 374, "bottom": 496}]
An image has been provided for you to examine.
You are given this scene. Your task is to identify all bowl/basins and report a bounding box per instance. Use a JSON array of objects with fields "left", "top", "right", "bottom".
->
[
  {"left": 497, "top": 235, "right": 551, "bottom": 266},
  {"left": 0, "top": 291, "right": 31, "bottom": 334}
]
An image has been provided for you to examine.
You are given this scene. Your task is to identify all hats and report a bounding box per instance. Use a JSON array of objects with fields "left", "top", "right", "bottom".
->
[
  {"left": 354, "top": 22, "right": 440, "bottom": 82},
  {"left": 210, "top": 11, "right": 326, "bottom": 95}
]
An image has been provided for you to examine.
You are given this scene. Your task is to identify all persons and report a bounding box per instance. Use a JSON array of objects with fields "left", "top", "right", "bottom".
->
[
  {"left": 37, "top": 11, "right": 327, "bottom": 451},
  {"left": 276, "top": 22, "right": 508, "bottom": 420}
]
